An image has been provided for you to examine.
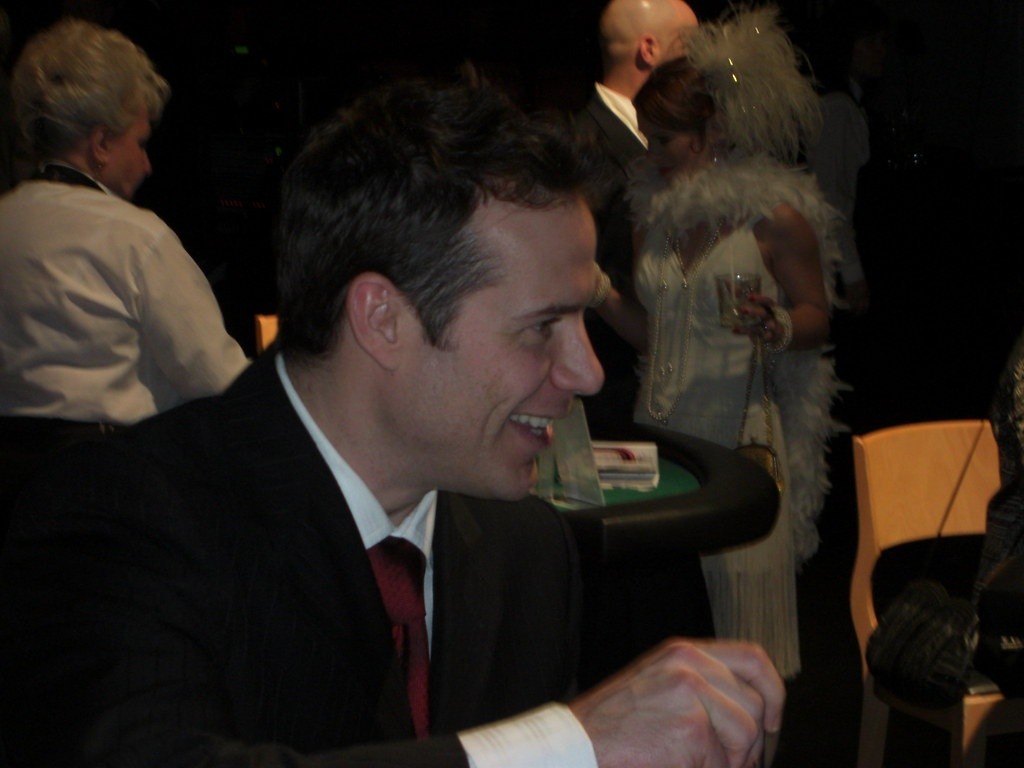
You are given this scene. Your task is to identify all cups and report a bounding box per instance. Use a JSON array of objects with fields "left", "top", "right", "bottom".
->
[{"left": 715, "top": 272, "right": 762, "bottom": 329}]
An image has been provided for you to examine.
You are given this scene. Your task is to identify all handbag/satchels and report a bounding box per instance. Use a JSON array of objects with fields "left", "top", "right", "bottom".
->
[{"left": 867, "top": 580, "right": 983, "bottom": 713}]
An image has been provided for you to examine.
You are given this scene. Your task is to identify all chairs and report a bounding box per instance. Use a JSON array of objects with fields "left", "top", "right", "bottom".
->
[{"left": 850, "top": 419, "right": 1024, "bottom": 768}]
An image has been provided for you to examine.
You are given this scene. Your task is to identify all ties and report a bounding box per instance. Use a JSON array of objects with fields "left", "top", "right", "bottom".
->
[{"left": 367, "top": 536, "right": 429, "bottom": 742}]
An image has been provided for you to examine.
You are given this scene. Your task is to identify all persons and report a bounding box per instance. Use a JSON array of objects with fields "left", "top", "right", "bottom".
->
[
  {"left": 552, "top": 0, "right": 700, "bottom": 443},
  {"left": 973, "top": 328, "right": 1024, "bottom": 702},
  {"left": 587, "top": 9, "right": 855, "bottom": 681},
  {"left": 0, "top": 13, "right": 254, "bottom": 426},
  {"left": 0, "top": 69, "right": 787, "bottom": 768},
  {"left": 799, "top": 0, "right": 891, "bottom": 431}
]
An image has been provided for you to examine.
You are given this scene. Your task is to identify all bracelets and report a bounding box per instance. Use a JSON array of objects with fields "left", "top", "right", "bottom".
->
[
  {"left": 757, "top": 307, "right": 793, "bottom": 354},
  {"left": 588, "top": 261, "right": 611, "bottom": 309}
]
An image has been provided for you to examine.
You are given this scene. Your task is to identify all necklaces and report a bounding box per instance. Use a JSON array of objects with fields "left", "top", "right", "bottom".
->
[{"left": 647, "top": 219, "right": 722, "bottom": 420}]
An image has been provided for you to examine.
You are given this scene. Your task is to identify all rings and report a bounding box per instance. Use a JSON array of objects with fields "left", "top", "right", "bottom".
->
[{"left": 762, "top": 323, "right": 768, "bottom": 332}]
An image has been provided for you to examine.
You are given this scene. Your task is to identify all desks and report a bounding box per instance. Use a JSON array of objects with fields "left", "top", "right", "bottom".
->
[{"left": 549, "top": 418, "right": 782, "bottom": 702}]
